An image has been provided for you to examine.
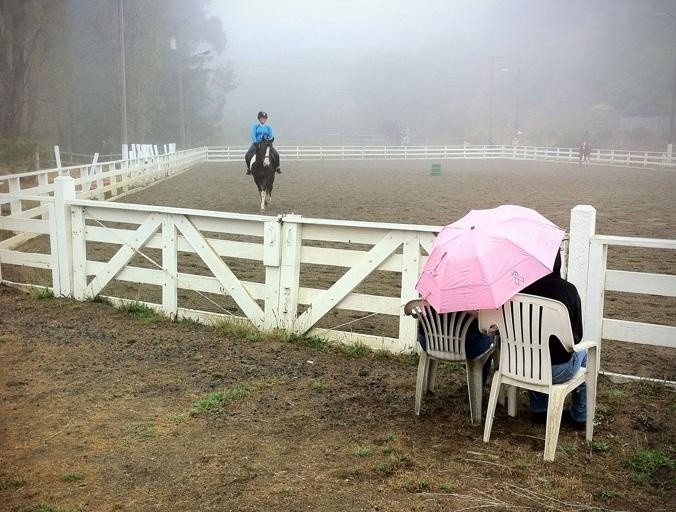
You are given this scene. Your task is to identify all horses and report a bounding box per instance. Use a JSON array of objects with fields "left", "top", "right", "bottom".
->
[
  {"left": 250, "top": 135, "right": 275, "bottom": 213},
  {"left": 580, "top": 137, "right": 592, "bottom": 168}
]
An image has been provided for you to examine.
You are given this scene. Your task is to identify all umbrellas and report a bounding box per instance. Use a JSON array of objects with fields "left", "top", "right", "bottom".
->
[{"left": 415, "top": 203, "right": 568, "bottom": 316}]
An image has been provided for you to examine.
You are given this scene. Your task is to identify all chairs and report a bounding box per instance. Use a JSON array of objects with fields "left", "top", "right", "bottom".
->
[
  {"left": 404, "top": 299, "right": 505, "bottom": 427},
  {"left": 479, "top": 294, "right": 599, "bottom": 462}
]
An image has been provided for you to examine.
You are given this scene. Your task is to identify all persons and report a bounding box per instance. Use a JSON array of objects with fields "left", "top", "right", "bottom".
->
[
  {"left": 518, "top": 249, "right": 589, "bottom": 424},
  {"left": 245, "top": 111, "right": 282, "bottom": 175},
  {"left": 416, "top": 301, "right": 494, "bottom": 404},
  {"left": 582, "top": 130, "right": 592, "bottom": 144}
]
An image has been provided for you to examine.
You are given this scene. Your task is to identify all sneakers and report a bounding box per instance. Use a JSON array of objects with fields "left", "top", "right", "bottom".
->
[
  {"left": 245, "top": 169, "right": 251, "bottom": 175},
  {"left": 530, "top": 406, "right": 588, "bottom": 430},
  {"left": 276, "top": 168, "right": 281, "bottom": 174}
]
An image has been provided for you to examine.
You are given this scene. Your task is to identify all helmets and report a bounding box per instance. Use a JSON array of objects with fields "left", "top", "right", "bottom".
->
[{"left": 257, "top": 111, "right": 268, "bottom": 120}]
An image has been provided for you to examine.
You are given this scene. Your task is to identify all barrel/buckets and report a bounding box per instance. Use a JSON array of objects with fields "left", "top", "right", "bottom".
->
[{"left": 431, "top": 164, "right": 442, "bottom": 178}]
